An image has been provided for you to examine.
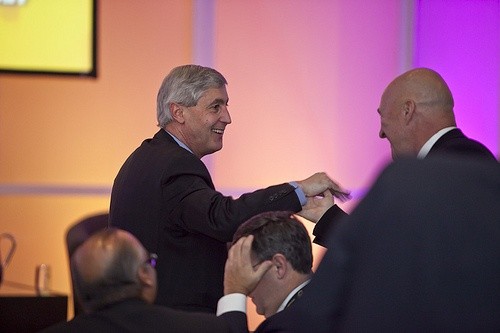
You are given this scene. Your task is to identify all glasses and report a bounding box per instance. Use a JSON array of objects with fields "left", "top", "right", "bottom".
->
[{"left": 138, "top": 253, "right": 158, "bottom": 272}]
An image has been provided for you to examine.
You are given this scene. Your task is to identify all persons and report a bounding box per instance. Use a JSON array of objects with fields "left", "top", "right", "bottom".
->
[
  {"left": 214, "top": 212, "right": 319, "bottom": 333},
  {"left": 107, "top": 64, "right": 353, "bottom": 312},
  {"left": 48, "top": 228, "right": 224, "bottom": 333},
  {"left": 295, "top": 68, "right": 500, "bottom": 333}
]
